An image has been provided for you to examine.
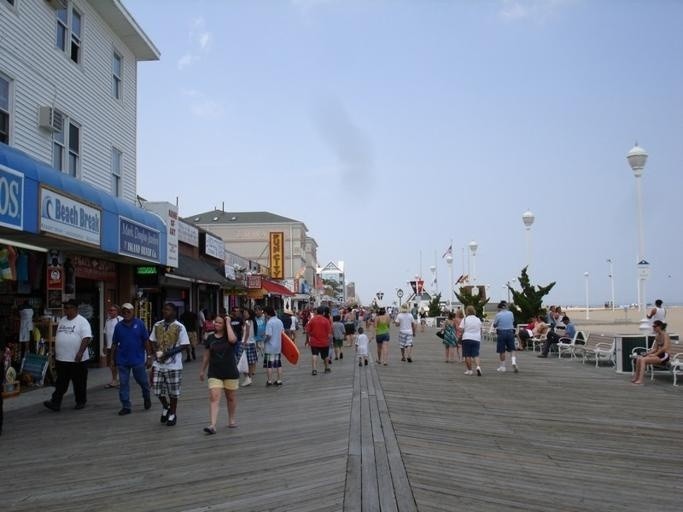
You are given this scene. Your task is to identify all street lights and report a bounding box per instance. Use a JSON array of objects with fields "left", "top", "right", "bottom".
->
[
  {"left": 446, "top": 253, "right": 453, "bottom": 312},
  {"left": 522, "top": 208, "right": 536, "bottom": 272},
  {"left": 415, "top": 273, "right": 420, "bottom": 295},
  {"left": 584, "top": 270, "right": 591, "bottom": 320},
  {"left": 467, "top": 239, "right": 479, "bottom": 285},
  {"left": 607, "top": 256, "right": 617, "bottom": 313},
  {"left": 625, "top": 140, "right": 651, "bottom": 336},
  {"left": 373, "top": 286, "right": 398, "bottom": 311},
  {"left": 429, "top": 265, "right": 437, "bottom": 293}
]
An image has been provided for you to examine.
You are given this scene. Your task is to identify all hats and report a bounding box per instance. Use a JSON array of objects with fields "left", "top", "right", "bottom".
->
[
  {"left": 560, "top": 317, "right": 569, "bottom": 321},
  {"left": 62, "top": 298, "right": 78, "bottom": 307},
  {"left": 120, "top": 302, "right": 134, "bottom": 310}
]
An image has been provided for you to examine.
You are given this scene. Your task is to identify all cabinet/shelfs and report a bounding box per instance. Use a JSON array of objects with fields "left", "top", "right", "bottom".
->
[{"left": 32, "top": 320, "right": 59, "bottom": 385}]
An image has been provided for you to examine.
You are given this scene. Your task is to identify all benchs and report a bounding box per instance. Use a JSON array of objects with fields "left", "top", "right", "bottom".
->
[
  {"left": 571, "top": 330, "right": 616, "bottom": 369},
  {"left": 525, "top": 327, "right": 552, "bottom": 352},
  {"left": 481, "top": 320, "right": 498, "bottom": 344},
  {"left": 629, "top": 339, "right": 683, "bottom": 386}
]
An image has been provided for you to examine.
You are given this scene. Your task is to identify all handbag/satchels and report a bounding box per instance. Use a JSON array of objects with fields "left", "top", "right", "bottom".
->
[{"left": 436, "top": 329, "right": 444, "bottom": 339}]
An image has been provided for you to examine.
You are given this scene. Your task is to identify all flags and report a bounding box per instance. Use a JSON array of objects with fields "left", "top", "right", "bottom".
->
[
  {"left": 440, "top": 245, "right": 451, "bottom": 259},
  {"left": 409, "top": 280, "right": 416, "bottom": 294},
  {"left": 416, "top": 280, "right": 425, "bottom": 294}
]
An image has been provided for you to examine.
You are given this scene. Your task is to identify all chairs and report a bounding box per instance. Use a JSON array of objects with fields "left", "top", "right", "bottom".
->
[{"left": 556, "top": 331, "right": 587, "bottom": 360}]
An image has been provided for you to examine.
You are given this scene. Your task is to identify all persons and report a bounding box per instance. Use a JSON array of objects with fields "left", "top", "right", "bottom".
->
[
  {"left": 148, "top": 302, "right": 191, "bottom": 427},
  {"left": 299, "top": 304, "right": 392, "bottom": 374},
  {"left": 644, "top": 298, "right": 667, "bottom": 335},
  {"left": 395, "top": 304, "right": 415, "bottom": 363},
  {"left": 630, "top": 319, "right": 671, "bottom": 386},
  {"left": 15, "top": 299, "right": 36, "bottom": 372},
  {"left": 110, "top": 301, "right": 153, "bottom": 416},
  {"left": 442, "top": 305, "right": 482, "bottom": 376},
  {"left": 411, "top": 303, "right": 426, "bottom": 336},
  {"left": 492, "top": 302, "right": 519, "bottom": 372},
  {"left": 264, "top": 306, "right": 284, "bottom": 385},
  {"left": 282, "top": 310, "right": 298, "bottom": 342},
  {"left": 517, "top": 306, "right": 576, "bottom": 358},
  {"left": 182, "top": 302, "right": 215, "bottom": 362},
  {"left": 230, "top": 305, "right": 267, "bottom": 384},
  {"left": 198, "top": 314, "right": 240, "bottom": 436},
  {"left": 42, "top": 298, "right": 95, "bottom": 414},
  {"left": 102, "top": 303, "right": 125, "bottom": 390}
]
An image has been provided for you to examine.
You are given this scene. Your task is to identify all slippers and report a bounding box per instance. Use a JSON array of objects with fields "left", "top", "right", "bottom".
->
[
  {"left": 204, "top": 427, "right": 216, "bottom": 434},
  {"left": 104, "top": 384, "right": 116, "bottom": 389},
  {"left": 116, "top": 385, "right": 120, "bottom": 389}
]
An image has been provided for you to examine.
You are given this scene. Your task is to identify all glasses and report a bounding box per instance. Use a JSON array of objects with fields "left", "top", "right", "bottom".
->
[
  {"left": 121, "top": 310, "right": 130, "bottom": 314},
  {"left": 64, "top": 306, "right": 75, "bottom": 309},
  {"left": 108, "top": 309, "right": 116, "bottom": 311}
]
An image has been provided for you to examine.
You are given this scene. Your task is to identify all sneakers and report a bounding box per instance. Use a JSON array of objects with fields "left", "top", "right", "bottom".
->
[
  {"left": 464, "top": 371, "right": 472, "bottom": 375},
  {"left": 167, "top": 414, "right": 177, "bottom": 426},
  {"left": 161, "top": 404, "right": 170, "bottom": 423},
  {"left": 364, "top": 359, "right": 368, "bottom": 366},
  {"left": 511, "top": 362, "right": 518, "bottom": 372},
  {"left": 407, "top": 357, "right": 411, "bottom": 362},
  {"left": 476, "top": 366, "right": 480, "bottom": 376},
  {"left": 144, "top": 399, "right": 151, "bottom": 409},
  {"left": 241, "top": 380, "right": 252, "bottom": 387},
  {"left": 496, "top": 368, "right": 505, "bottom": 372},
  {"left": 118, "top": 408, "right": 130, "bottom": 415},
  {"left": 340, "top": 352, "right": 343, "bottom": 360}
]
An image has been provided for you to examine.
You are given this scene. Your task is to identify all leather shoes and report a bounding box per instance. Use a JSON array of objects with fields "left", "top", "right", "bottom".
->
[
  {"left": 75, "top": 404, "right": 85, "bottom": 410},
  {"left": 44, "top": 401, "right": 60, "bottom": 411}
]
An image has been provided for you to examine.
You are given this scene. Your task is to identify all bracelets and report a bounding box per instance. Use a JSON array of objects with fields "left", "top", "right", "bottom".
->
[
  {"left": 78, "top": 351, "right": 83, "bottom": 357},
  {"left": 145, "top": 354, "right": 153, "bottom": 359}
]
